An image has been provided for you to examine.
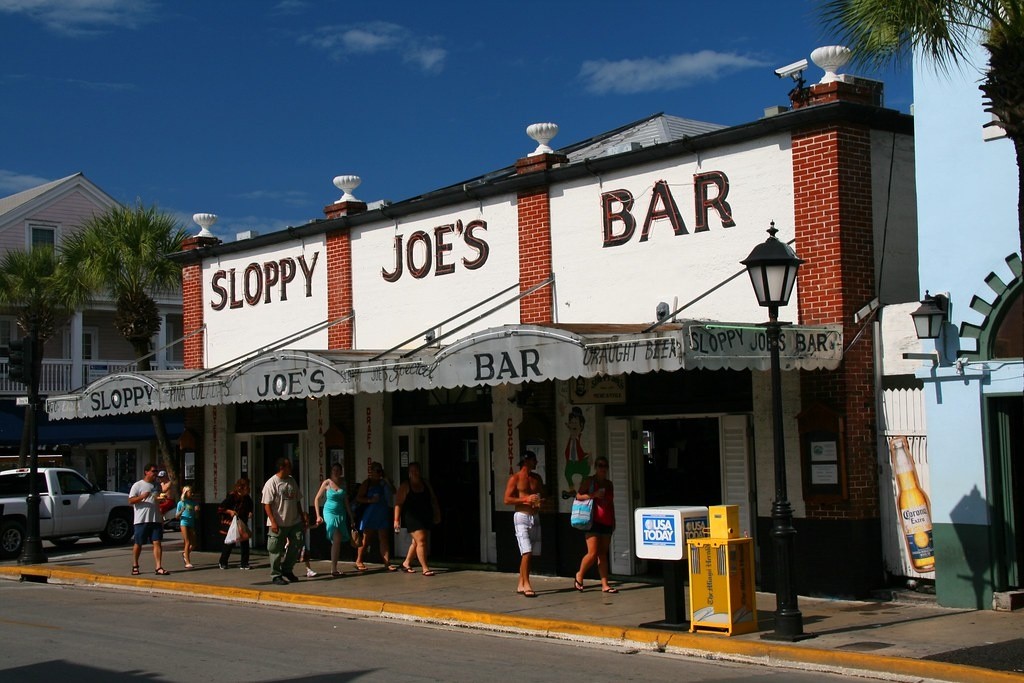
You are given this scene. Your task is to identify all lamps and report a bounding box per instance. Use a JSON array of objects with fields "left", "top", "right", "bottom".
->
[{"left": 909, "top": 288, "right": 951, "bottom": 338}]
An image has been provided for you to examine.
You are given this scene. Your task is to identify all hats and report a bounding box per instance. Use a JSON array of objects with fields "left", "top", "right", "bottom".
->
[
  {"left": 157, "top": 471, "right": 165, "bottom": 477},
  {"left": 181, "top": 486, "right": 192, "bottom": 502}
]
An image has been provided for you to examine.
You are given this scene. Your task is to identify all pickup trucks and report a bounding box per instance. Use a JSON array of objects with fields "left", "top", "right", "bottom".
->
[{"left": 0, "top": 468, "right": 136, "bottom": 561}]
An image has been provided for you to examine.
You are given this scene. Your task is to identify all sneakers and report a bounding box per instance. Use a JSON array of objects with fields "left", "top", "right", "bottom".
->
[{"left": 306, "top": 570, "right": 317, "bottom": 577}]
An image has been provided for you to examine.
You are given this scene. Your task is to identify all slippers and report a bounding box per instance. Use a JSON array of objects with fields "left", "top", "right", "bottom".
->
[
  {"left": 575, "top": 575, "right": 583, "bottom": 591},
  {"left": 353, "top": 565, "right": 368, "bottom": 570},
  {"left": 385, "top": 565, "right": 397, "bottom": 571},
  {"left": 422, "top": 571, "right": 434, "bottom": 576},
  {"left": 601, "top": 588, "right": 618, "bottom": 593},
  {"left": 517, "top": 590, "right": 536, "bottom": 596},
  {"left": 402, "top": 568, "right": 416, "bottom": 573}
]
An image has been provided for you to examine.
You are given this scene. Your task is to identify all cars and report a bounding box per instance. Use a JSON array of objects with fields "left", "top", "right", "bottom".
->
[{"left": 162, "top": 506, "right": 181, "bottom": 532}]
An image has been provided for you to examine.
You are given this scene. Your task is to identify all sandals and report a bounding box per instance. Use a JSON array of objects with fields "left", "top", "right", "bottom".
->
[
  {"left": 155, "top": 568, "right": 168, "bottom": 575},
  {"left": 132, "top": 566, "right": 139, "bottom": 574}
]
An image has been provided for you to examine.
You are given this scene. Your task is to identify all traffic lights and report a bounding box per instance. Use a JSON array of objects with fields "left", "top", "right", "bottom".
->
[{"left": 8, "top": 336, "right": 30, "bottom": 384}]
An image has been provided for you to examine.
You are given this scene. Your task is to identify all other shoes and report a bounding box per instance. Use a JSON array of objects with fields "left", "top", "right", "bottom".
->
[
  {"left": 240, "top": 565, "right": 250, "bottom": 570},
  {"left": 517, "top": 451, "right": 534, "bottom": 466},
  {"left": 286, "top": 574, "right": 297, "bottom": 582},
  {"left": 219, "top": 563, "right": 227, "bottom": 569},
  {"left": 185, "top": 565, "right": 193, "bottom": 568},
  {"left": 182, "top": 552, "right": 186, "bottom": 563},
  {"left": 273, "top": 577, "right": 288, "bottom": 585}
]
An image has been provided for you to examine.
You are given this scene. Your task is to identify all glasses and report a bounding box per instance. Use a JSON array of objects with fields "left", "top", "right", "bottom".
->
[
  {"left": 596, "top": 464, "right": 608, "bottom": 467},
  {"left": 152, "top": 470, "right": 158, "bottom": 472}
]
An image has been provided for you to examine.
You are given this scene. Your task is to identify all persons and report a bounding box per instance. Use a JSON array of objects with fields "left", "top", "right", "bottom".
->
[
  {"left": 394, "top": 461, "right": 441, "bottom": 576},
  {"left": 218, "top": 478, "right": 255, "bottom": 570},
  {"left": 176, "top": 486, "right": 201, "bottom": 568},
  {"left": 355, "top": 462, "right": 397, "bottom": 573},
  {"left": 575, "top": 457, "right": 618, "bottom": 593},
  {"left": 128, "top": 462, "right": 169, "bottom": 575},
  {"left": 261, "top": 457, "right": 321, "bottom": 584},
  {"left": 314, "top": 462, "right": 355, "bottom": 578},
  {"left": 503, "top": 450, "right": 550, "bottom": 597},
  {"left": 157, "top": 471, "right": 177, "bottom": 516}
]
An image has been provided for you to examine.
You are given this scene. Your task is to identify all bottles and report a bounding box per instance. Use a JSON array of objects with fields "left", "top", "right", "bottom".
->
[{"left": 886, "top": 437, "right": 938, "bottom": 574}]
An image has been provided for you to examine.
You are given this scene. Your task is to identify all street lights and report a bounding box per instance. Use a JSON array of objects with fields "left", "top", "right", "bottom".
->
[{"left": 738, "top": 217, "right": 818, "bottom": 644}]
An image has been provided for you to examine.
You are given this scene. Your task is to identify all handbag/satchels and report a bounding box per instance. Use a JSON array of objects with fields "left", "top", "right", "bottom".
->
[
  {"left": 571, "top": 476, "right": 594, "bottom": 530},
  {"left": 224, "top": 515, "right": 251, "bottom": 545},
  {"left": 356, "top": 505, "right": 375, "bottom": 531},
  {"left": 351, "top": 529, "right": 361, "bottom": 548}
]
